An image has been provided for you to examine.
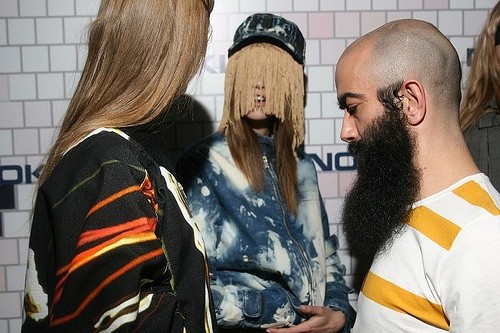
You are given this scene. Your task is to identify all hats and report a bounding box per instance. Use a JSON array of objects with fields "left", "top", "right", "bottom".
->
[{"left": 228, "top": 12, "right": 306, "bottom": 66}]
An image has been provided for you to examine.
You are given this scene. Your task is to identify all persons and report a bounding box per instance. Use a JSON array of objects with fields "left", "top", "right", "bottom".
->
[
  {"left": 188, "top": 13, "right": 356, "bottom": 333},
  {"left": 459, "top": 0, "right": 500, "bottom": 193},
  {"left": 335, "top": 19, "right": 500, "bottom": 333},
  {"left": 21, "top": 0, "right": 217, "bottom": 333}
]
